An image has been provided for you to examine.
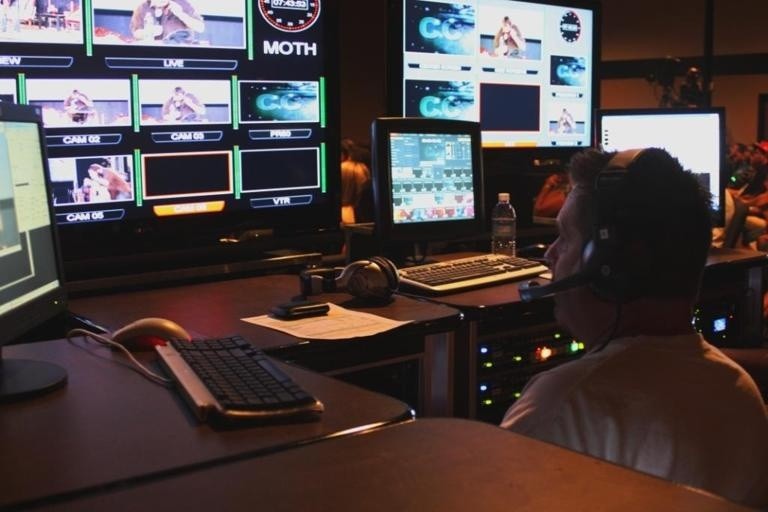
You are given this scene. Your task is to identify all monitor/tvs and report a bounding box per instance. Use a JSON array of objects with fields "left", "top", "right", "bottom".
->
[
  {"left": 369, "top": 116, "right": 487, "bottom": 241},
  {"left": 597, "top": 106, "right": 726, "bottom": 228},
  {"left": 385, "top": 0, "right": 603, "bottom": 160},
  {"left": 0, "top": 102, "right": 70, "bottom": 402},
  {"left": 0, "top": 0, "right": 342, "bottom": 296}
]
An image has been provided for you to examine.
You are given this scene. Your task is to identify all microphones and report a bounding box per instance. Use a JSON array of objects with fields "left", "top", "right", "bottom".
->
[{"left": 513, "top": 240, "right": 606, "bottom": 305}]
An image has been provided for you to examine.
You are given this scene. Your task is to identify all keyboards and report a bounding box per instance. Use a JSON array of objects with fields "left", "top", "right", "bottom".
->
[
  {"left": 154, "top": 334, "right": 326, "bottom": 424},
  {"left": 397, "top": 254, "right": 549, "bottom": 295}
]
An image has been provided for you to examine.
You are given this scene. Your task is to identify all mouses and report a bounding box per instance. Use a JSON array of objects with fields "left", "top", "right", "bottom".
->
[
  {"left": 111, "top": 316, "right": 191, "bottom": 346},
  {"left": 520, "top": 243, "right": 547, "bottom": 257}
]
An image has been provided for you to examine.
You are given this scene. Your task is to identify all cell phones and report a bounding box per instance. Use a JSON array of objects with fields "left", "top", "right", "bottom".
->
[{"left": 264, "top": 301, "right": 331, "bottom": 324}]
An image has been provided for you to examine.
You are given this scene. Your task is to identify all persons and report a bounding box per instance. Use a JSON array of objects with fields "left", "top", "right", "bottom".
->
[
  {"left": 129, "top": 0, "right": 205, "bottom": 45},
  {"left": 0, "top": 0, "right": 21, "bottom": 35},
  {"left": 557, "top": 107, "right": 577, "bottom": 133},
  {"left": 63, "top": 88, "right": 94, "bottom": 107},
  {"left": 491, "top": 147, "right": 768, "bottom": 512},
  {"left": 83, "top": 163, "right": 132, "bottom": 200},
  {"left": 493, "top": 16, "right": 525, "bottom": 59},
  {"left": 162, "top": 87, "right": 206, "bottom": 122},
  {"left": 534, "top": 173, "right": 571, "bottom": 216}
]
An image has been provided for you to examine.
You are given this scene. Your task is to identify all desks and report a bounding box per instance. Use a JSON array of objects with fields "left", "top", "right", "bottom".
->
[
  {"left": 0, "top": 271, "right": 463, "bottom": 510},
  {"left": 390, "top": 233, "right": 767, "bottom": 416},
  {"left": 3, "top": 415, "right": 750, "bottom": 512}
]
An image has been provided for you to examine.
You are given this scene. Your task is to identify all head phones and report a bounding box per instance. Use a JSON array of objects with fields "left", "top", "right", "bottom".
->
[
  {"left": 582, "top": 147, "right": 661, "bottom": 303},
  {"left": 300, "top": 256, "right": 399, "bottom": 301}
]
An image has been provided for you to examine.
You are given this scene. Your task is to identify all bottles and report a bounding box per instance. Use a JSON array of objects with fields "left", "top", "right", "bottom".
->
[{"left": 489, "top": 193, "right": 517, "bottom": 257}]
